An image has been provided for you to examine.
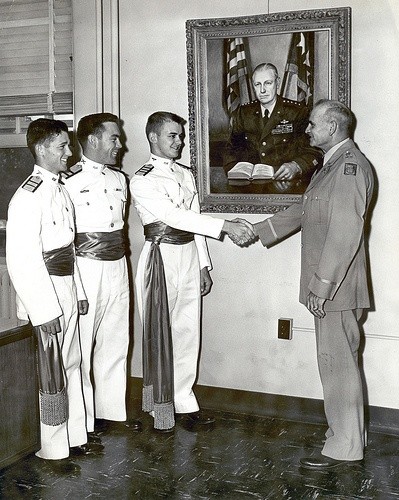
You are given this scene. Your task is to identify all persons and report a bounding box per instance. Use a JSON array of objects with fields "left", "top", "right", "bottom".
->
[
  {"left": 223, "top": 63, "right": 322, "bottom": 182},
  {"left": 228, "top": 99, "right": 374, "bottom": 470},
  {"left": 58, "top": 113, "right": 143, "bottom": 443},
  {"left": 5, "top": 119, "right": 105, "bottom": 475},
  {"left": 129, "top": 111, "right": 253, "bottom": 433}
]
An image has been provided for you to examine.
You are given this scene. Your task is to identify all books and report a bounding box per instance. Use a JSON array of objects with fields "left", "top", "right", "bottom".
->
[{"left": 227, "top": 161, "right": 274, "bottom": 182}]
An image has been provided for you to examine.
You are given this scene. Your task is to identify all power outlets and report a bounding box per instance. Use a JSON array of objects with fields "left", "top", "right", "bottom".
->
[{"left": 277, "top": 318, "right": 293, "bottom": 341}]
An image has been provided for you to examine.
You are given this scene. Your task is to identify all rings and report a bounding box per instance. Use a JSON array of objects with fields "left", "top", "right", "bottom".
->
[
  {"left": 313, "top": 308, "right": 318, "bottom": 311},
  {"left": 284, "top": 176, "right": 287, "bottom": 180}
]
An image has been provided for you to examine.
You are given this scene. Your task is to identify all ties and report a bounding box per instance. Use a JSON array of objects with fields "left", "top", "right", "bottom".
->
[{"left": 315, "top": 157, "right": 325, "bottom": 174}]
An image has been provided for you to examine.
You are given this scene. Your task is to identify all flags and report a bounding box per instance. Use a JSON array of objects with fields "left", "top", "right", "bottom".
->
[
  {"left": 221, "top": 37, "right": 257, "bottom": 117},
  {"left": 281, "top": 33, "right": 314, "bottom": 107}
]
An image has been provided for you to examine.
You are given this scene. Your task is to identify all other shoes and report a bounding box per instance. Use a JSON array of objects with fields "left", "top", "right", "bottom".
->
[
  {"left": 43, "top": 457, "right": 81, "bottom": 474},
  {"left": 95, "top": 419, "right": 143, "bottom": 429},
  {"left": 183, "top": 411, "right": 216, "bottom": 424},
  {"left": 301, "top": 433, "right": 328, "bottom": 448},
  {"left": 300, "top": 451, "right": 360, "bottom": 470},
  {"left": 70, "top": 444, "right": 105, "bottom": 455},
  {"left": 87, "top": 433, "right": 102, "bottom": 443}
]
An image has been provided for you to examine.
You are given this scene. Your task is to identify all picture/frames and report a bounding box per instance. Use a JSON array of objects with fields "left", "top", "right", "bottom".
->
[{"left": 187, "top": 6, "right": 352, "bottom": 214}]
